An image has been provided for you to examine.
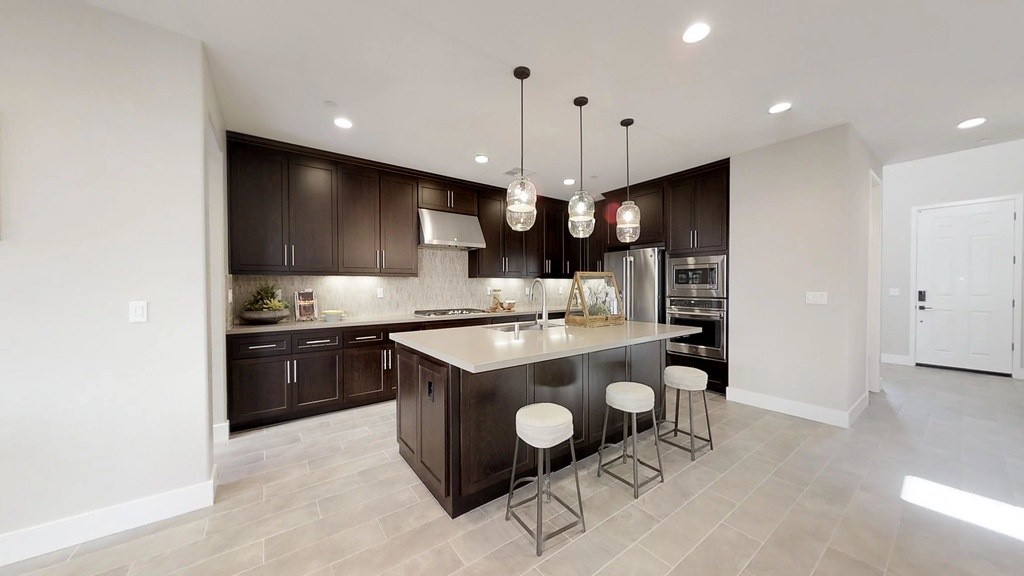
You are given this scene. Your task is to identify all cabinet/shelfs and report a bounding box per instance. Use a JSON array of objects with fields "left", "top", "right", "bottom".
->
[
  {"left": 338, "top": 154, "right": 417, "bottom": 277},
  {"left": 564, "top": 201, "right": 585, "bottom": 279},
  {"left": 342, "top": 323, "right": 426, "bottom": 411},
  {"left": 468, "top": 181, "right": 529, "bottom": 279},
  {"left": 524, "top": 194, "right": 565, "bottom": 279},
  {"left": 664, "top": 157, "right": 730, "bottom": 259},
  {"left": 584, "top": 199, "right": 605, "bottom": 272},
  {"left": 226, "top": 129, "right": 338, "bottom": 277},
  {"left": 598, "top": 175, "right": 666, "bottom": 253},
  {"left": 418, "top": 170, "right": 478, "bottom": 216},
  {"left": 225, "top": 327, "right": 343, "bottom": 436}
]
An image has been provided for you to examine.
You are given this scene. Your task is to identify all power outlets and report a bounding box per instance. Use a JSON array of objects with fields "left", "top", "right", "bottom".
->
[
  {"left": 558, "top": 287, "right": 563, "bottom": 295},
  {"left": 376, "top": 287, "right": 384, "bottom": 298},
  {"left": 487, "top": 286, "right": 493, "bottom": 296},
  {"left": 274, "top": 289, "right": 281, "bottom": 300},
  {"left": 228, "top": 289, "right": 233, "bottom": 303},
  {"left": 806, "top": 291, "right": 828, "bottom": 305},
  {"left": 526, "top": 287, "right": 529, "bottom": 295}
]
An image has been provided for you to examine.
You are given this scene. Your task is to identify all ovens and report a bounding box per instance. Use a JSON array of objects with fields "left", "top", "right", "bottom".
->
[{"left": 665, "top": 255, "right": 726, "bottom": 360}]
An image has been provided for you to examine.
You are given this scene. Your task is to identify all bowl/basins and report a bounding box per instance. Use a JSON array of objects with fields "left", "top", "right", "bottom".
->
[{"left": 499, "top": 302, "right": 515, "bottom": 310}]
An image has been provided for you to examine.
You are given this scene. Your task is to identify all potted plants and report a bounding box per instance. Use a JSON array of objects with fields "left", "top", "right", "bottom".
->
[{"left": 240, "top": 282, "right": 291, "bottom": 326}]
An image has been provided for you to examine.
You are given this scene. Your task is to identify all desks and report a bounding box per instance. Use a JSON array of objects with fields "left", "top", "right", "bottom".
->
[{"left": 388, "top": 316, "right": 703, "bottom": 521}]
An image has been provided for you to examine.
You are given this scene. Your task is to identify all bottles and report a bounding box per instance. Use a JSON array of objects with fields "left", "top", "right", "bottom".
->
[{"left": 492, "top": 290, "right": 501, "bottom": 311}]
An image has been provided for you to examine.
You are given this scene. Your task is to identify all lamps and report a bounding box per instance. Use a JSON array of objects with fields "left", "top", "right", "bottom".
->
[
  {"left": 615, "top": 118, "right": 642, "bottom": 244},
  {"left": 505, "top": 65, "right": 538, "bottom": 232},
  {"left": 567, "top": 95, "right": 597, "bottom": 239}
]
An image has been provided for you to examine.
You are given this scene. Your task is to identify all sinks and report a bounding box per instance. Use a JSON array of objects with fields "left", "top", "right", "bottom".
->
[{"left": 481, "top": 322, "right": 566, "bottom": 333}]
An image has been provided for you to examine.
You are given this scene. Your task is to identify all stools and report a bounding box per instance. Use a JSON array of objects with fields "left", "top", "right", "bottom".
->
[
  {"left": 597, "top": 381, "right": 665, "bottom": 500},
  {"left": 504, "top": 402, "right": 586, "bottom": 557},
  {"left": 654, "top": 365, "right": 713, "bottom": 462}
]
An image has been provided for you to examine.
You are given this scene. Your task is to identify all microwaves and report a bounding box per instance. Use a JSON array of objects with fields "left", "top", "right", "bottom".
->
[{"left": 419, "top": 209, "right": 486, "bottom": 252}]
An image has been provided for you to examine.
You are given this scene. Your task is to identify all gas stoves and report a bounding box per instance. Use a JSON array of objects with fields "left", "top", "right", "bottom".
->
[{"left": 414, "top": 307, "right": 491, "bottom": 318}]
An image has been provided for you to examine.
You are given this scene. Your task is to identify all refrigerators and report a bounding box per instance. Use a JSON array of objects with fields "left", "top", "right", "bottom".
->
[{"left": 603, "top": 247, "right": 665, "bottom": 324}]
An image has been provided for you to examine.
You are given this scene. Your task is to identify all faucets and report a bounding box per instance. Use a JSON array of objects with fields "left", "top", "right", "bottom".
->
[{"left": 529, "top": 277, "right": 548, "bottom": 330}]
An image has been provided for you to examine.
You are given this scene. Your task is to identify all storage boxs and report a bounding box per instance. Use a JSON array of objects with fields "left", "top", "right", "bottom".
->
[
  {"left": 564, "top": 271, "right": 625, "bottom": 327},
  {"left": 293, "top": 290, "right": 318, "bottom": 322}
]
